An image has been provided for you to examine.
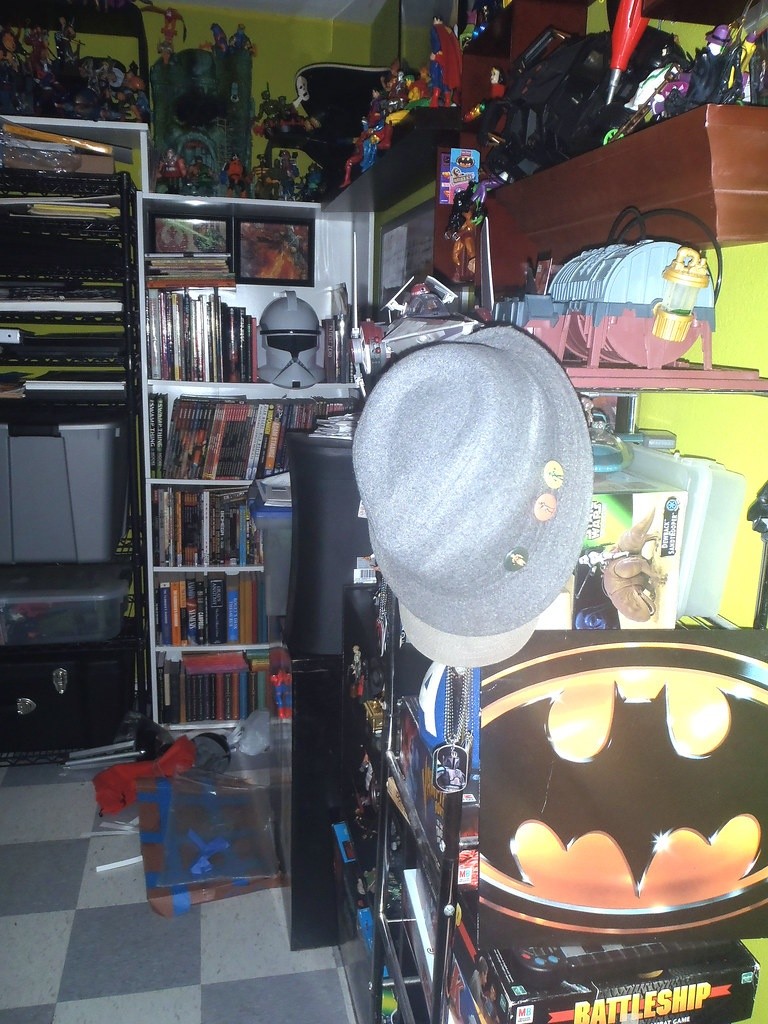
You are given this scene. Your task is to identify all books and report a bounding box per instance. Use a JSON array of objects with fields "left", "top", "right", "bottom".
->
[
  {"left": 0, "top": 194, "right": 126, "bottom": 406},
  {"left": 144, "top": 252, "right": 237, "bottom": 289},
  {"left": 153, "top": 571, "right": 270, "bottom": 647},
  {"left": 145, "top": 289, "right": 258, "bottom": 383},
  {"left": 322, "top": 314, "right": 356, "bottom": 385},
  {"left": 151, "top": 486, "right": 264, "bottom": 567},
  {"left": 0, "top": 121, "right": 134, "bottom": 175},
  {"left": 156, "top": 651, "right": 272, "bottom": 724},
  {"left": 149, "top": 388, "right": 361, "bottom": 478}
]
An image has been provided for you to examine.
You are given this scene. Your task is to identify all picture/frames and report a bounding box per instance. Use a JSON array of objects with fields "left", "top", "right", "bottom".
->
[
  {"left": 234, "top": 217, "right": 315, "bottom": 287},
  {"left": 150, "top": 213, "right": 233, "bottom": 274}
]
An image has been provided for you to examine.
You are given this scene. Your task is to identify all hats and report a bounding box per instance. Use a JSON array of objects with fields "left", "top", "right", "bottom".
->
[
  {"left": 398, "top": 600, "right": 539, "bottom": 670},
  {"left": 350, "top": 323, "right": 594, "bottom": 637}
]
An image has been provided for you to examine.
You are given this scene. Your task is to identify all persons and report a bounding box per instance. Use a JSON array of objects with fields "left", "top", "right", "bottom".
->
[
  {"left": 445, "top": 148, "right": 515, "bottom": 282},
  {"left": 468, "top": 956, "right": 501, "bottom": 1023},
  {"left": 159, "top": 15, "right": 463, "bottom": 206}
]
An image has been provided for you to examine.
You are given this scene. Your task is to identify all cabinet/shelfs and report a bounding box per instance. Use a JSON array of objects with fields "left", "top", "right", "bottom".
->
[
  {"left": 333, "top": 579, "right": 768, "bottom": 1024},
  {"left": 0, "top": 167, "right": 145, "bottom": 759},
  {"left": 135, "top": 192, "right": 364, "bottom": 741}
]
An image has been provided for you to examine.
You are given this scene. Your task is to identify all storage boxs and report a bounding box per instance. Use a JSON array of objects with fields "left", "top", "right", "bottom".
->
[
  {"left": 572, "top": 469, "right": 688, "bottom": 629},
  {"left": 453, "top": 893, "right": 761, "bottom": 1024},
  {"left": 248, "top": 490, "right": 293, "bottom": 617},
  {"left": 402, "top": 868, "right": 440, "bottom": 1022},
  {"left": 0, "top": 568, "right": 129, "bottom": 646},
  {"left": 0, "top": 418, "right": 128, "bottom": 563}
]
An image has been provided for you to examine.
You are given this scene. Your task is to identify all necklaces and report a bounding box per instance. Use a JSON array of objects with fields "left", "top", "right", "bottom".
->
[
  {"left": 432, "top": 665, "right": 474, "bottom": 793},
  {"left": 377, "top": 576, "right": 388, "bottom": 657}
]
